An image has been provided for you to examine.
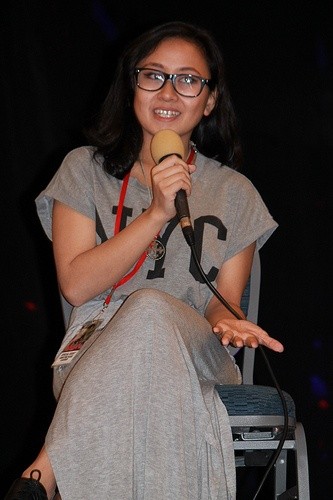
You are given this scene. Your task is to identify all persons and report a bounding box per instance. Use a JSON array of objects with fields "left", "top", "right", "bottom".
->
[{"left": 5, "top": 22, "right": 284, "bottom": 500}]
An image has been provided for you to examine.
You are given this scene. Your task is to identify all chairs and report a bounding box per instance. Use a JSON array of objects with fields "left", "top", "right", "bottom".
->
[{"left": 217, "top": 250, "right": 311, "bottom": 500}]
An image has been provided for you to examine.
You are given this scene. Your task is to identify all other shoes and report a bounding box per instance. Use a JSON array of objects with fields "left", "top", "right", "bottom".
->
[{"left": 4, "top": 475, "right": 59, "bottom": 500}]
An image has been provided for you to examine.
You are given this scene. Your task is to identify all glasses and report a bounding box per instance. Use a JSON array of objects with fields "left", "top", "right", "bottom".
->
[{"left": 133, "top": 67, "right": 215, "bottom": 99}]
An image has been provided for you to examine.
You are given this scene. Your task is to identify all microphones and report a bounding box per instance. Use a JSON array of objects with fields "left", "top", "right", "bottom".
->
[{"left": 151, "top": 130, "right": 195, "bottom": 246}]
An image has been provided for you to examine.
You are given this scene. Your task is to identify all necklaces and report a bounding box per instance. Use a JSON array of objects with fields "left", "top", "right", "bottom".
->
[{"left": 138, "top": 143, "right": 192, "bottom": 260}]
